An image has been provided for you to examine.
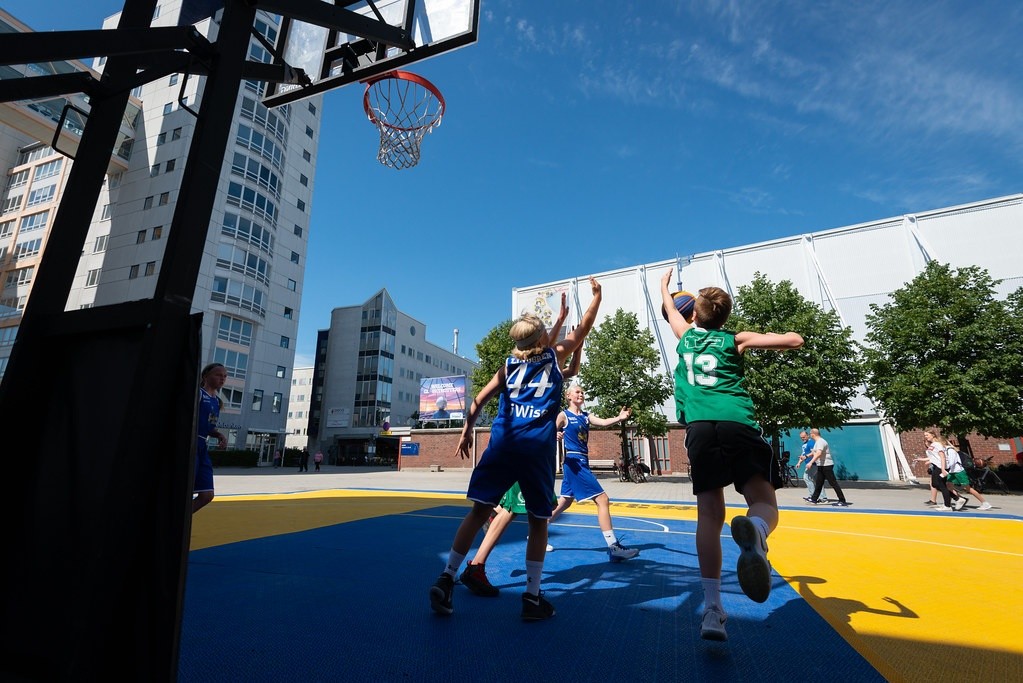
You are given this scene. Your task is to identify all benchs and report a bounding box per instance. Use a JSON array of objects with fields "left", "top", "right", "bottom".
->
[{"left": 589, "top": 460, "right": 615, "bottom": 467}]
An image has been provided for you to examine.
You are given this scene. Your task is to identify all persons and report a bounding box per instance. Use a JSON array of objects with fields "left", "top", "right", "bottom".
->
[
  {"left": 795, "top": 431, "right": 828, "bottom": 503},
  {"left": 660, "top": 267, "right": 804, "bottom": 641},
  {"left": 459, "top": 482, "right": 558, "bottom": 599},
  {"left": 190, "top": 363, "right": 228, "bottom": 516},
  {"left": 313, "top": 450, "right": 323, "bottom": 472},
  {"left": 524, "top": 385, "right": 639, "bottom": 563},
  {"left": 938, "top": 436, "right": 992, "bottom": 512},
  {"left": 274, "top": 449, "right": 281, "bottom": 468},
  {"left": 799, "top": 429, "right": 846, "bottom": 506},
  {"left": 912, "top": 438, "right": 953, "bottom": 504},
  {"left": 433, "top": 397, "right": 450, "bottom": 419},
  {"left": 429, "top": 277, "right": 603, "bottom": 620},
  {"left": 923, "top": 428, "right": 966, "bottom": 512},
  {"left": 298, "top": 448, "right": 310, "bottom": 472}
]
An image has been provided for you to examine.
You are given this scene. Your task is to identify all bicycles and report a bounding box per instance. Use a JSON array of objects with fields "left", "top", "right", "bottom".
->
[
  {"left": 617, "top": 452, "right": 648, "bottom": 483},
  {"left": 777, "top": 456, "right": 800, "bottom": 488},
  {"left": 963, "top": 455, "right": 1011, "bottom": 494},
  {"left": 681, "top": 461, "right": 693, "bottom": 482}
]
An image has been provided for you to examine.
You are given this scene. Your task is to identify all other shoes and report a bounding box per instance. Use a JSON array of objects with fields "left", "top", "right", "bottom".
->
[
  {"left": 922, "top": 500, "right": 937, "bottom": 508},
  {"left": 802, "top": 497, "right": 811, "bottom": 499},
  {"left": 817, "top": 498, "right": 829, "bottom": 504},
  {"left": 805, "top": 498, "right": 817, "bottom": 504},
  {"left": 832, "top": 501, "right": 846, "bottom": 507}
]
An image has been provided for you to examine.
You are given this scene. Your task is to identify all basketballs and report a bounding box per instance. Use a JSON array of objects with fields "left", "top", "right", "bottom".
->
[
  {"left": 975, "top": 458, "right": 987, "bottom": 469},
  {"left": 662, "top": 290, "right": 697, "bottom": 324}
]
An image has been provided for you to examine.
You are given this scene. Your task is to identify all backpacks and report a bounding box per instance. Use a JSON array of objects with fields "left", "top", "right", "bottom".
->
[{"left": 947, "top": 447, "right": 973, "bottom": 468}]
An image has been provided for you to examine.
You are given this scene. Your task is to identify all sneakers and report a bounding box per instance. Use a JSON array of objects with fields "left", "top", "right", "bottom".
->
[
  {"left": 459, "top": 560, "right": 498, "bottom": 595},
  {"left": 545, "top": 543, "right": 553, "bottom": 551},
  {"left": 935, "top": 505, "right": 952, "bottom": 511},
  {"left": 977, "top": 501, "right": 991, "bottom": 510},
  {"left": 699, "top": 603, "right": 727, "bottom": 640},
  {"left": 429, "top": 573, "right": 455, "bottom": 613},
  {"left": 608, "top": 533, "right": 637, "bottom": 562},
  {"left": 731, "top": 515, "right": 770, "bottom": 603},
  {"left": 954, "top": 497, "right": 966, "bottom": 509},
  {"left": 521, "top": 589, "right": 555, "bottom": 620}
]
